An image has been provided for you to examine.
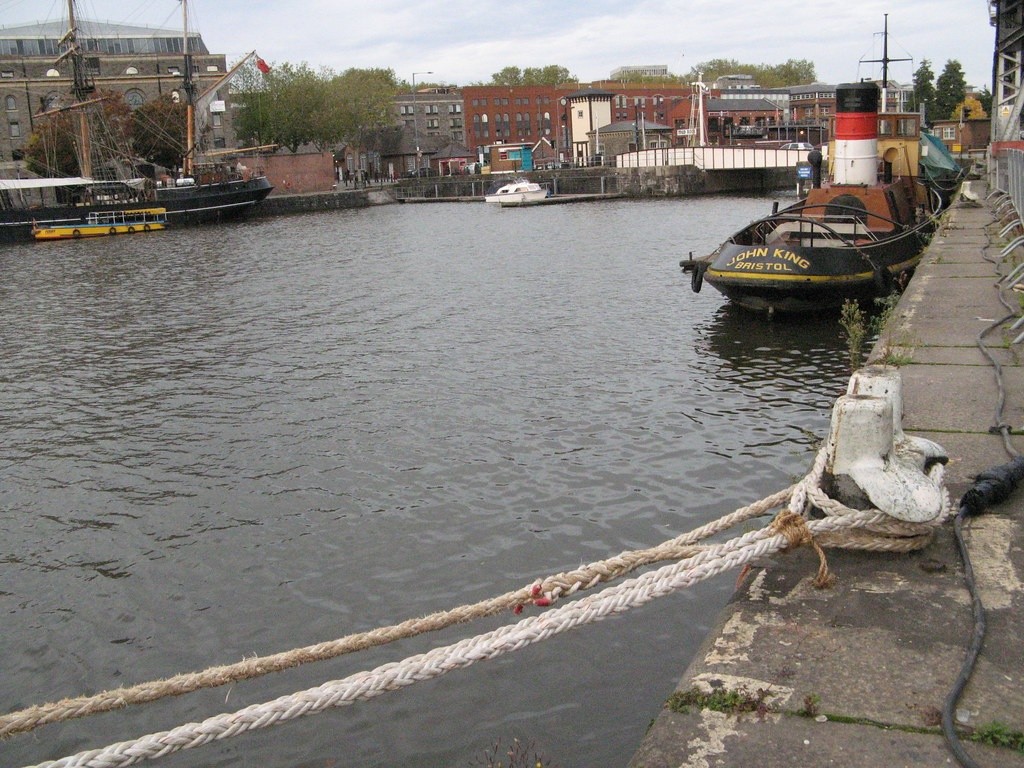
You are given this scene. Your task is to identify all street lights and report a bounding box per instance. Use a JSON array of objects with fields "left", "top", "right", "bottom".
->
[{"left": 412, "top": 71, "right": 434, "bottom": 179}]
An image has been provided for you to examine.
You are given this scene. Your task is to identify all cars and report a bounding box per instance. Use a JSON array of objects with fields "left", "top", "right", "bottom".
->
[
  {"left": 779, "top": 142, "right": 814, "bottom": 151},
  {"left": 411, "top": 167, "right": 435, "bottom": 178}
]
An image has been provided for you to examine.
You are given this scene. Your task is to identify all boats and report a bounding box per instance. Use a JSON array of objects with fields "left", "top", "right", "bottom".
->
[
  {"left": 484, "top": 177, "right": 548, "bottom": 204},
  {"left": 680, "top": 14, "right": 974, "bottom": 323}
]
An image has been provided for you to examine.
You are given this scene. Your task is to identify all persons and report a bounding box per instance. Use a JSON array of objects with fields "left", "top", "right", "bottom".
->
[{"left": 343, "top": 168, "right": 379, "bottom": 183}]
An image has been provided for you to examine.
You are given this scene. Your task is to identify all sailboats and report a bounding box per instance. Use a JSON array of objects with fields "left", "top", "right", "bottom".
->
[{"left": 0, "top": 0, "right": 275, "bottom": 240}]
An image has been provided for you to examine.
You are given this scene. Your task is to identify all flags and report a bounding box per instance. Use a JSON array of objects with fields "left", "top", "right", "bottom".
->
[{"left": 256, "top": 56, "right": 270, "bottom": 74}]
